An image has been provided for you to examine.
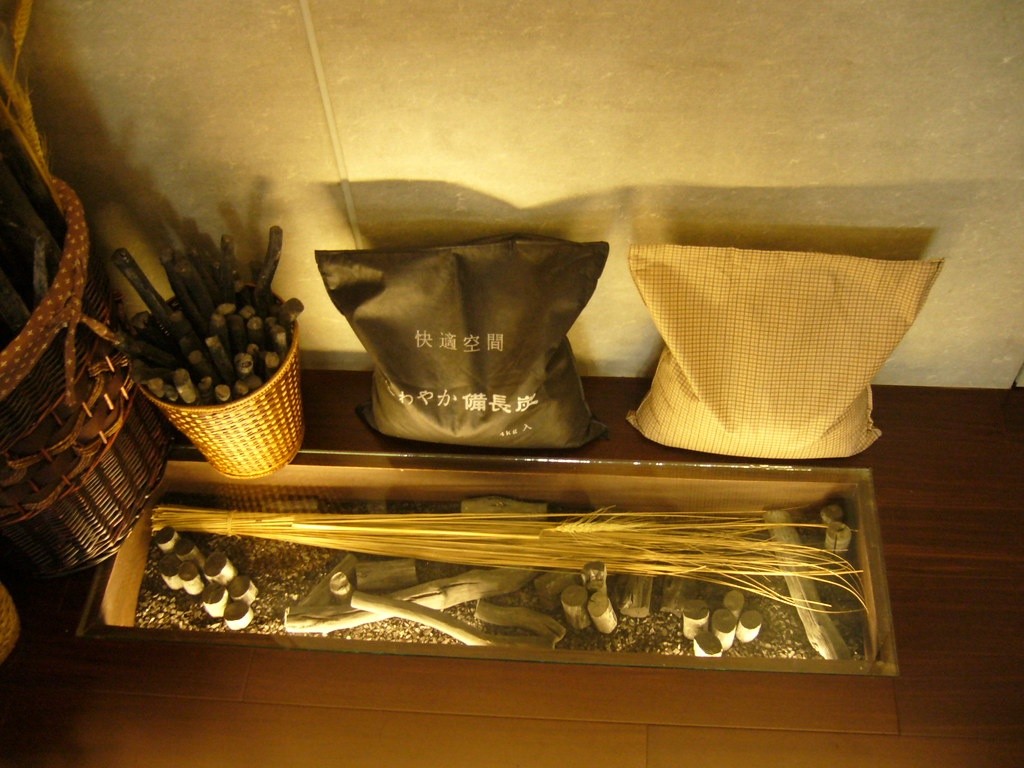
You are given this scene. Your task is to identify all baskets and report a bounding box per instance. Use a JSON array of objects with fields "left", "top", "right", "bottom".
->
[
  {"left": 0, "top": 583, "right": 21, "bottom": 663},
  {"left": 0, "top": 99, "right": 165, "bottom": 576},
  {"left": 128, "top": 279, "right": 305, "bottom": 480}
]
